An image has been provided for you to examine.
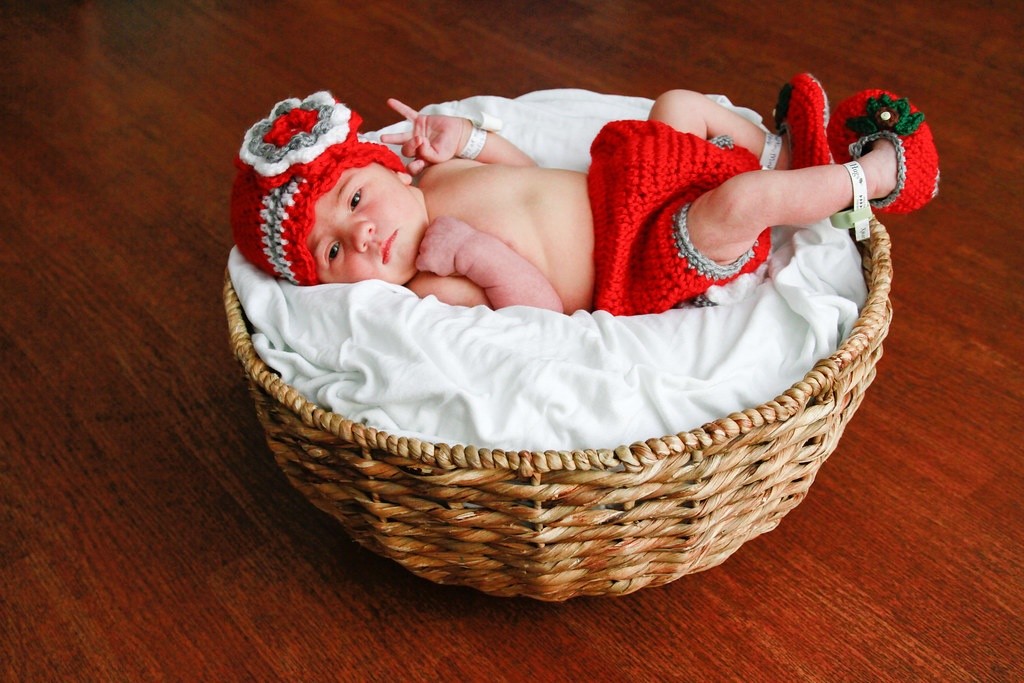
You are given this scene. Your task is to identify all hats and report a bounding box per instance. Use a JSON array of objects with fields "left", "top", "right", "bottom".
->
[{"left": 226, "top": 90, "right": 410, "bottom": 288}]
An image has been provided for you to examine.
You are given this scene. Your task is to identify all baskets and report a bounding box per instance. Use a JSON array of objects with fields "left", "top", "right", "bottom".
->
[{"left": 219, "top": 213, "right": 895, "bottom": 606}]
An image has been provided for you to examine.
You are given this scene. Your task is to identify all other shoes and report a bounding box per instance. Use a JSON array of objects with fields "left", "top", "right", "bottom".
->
[
  {"left": 825, "top": 88, "right": 940, "bottom": 216},
  {"left": 774, "top": 72, "right": 832, "bottom": 171}
]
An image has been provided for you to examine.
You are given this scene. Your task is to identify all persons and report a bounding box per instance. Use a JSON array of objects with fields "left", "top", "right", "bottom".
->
[{"left": 231, "top": 71, "right": 939, "bottom": 314}]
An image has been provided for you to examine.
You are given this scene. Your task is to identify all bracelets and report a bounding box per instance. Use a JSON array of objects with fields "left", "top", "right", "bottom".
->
[{"left": 456, "top": 112, "right": 502, "bottom": 160}]
